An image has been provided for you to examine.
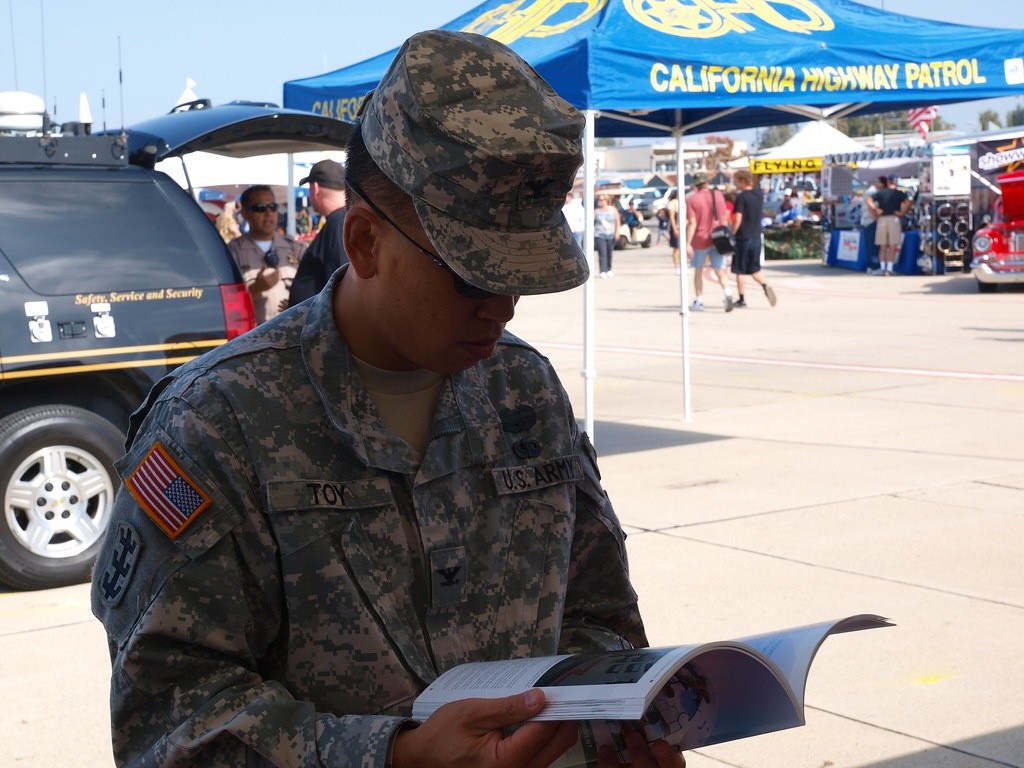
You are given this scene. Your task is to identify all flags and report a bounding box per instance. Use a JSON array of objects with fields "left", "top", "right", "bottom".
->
[{"left": 905, "top": 104, "right": 936, "bottom": 142}]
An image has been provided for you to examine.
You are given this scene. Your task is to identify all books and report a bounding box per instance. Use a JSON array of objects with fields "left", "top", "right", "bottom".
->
[{"left": 411, "top": 612, "right": 898, "bottom": 751}]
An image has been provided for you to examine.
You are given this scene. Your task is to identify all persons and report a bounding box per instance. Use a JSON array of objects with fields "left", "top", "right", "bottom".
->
[
  {"left": 595, "top": 193, "right": 621, "bottom": 278},
  {"left": 780, "top": 186, "right": 803, "bottom": 223},
  {"left": 620, "top": 199, "right": 644, "bottom": 237},
  {"left": 561, "top": 189, "right": 585, "bottom": 249},
  {"left": 684, "top": 173, "right": 733, "bottom": 312},
  {"left": 88, "top": 27, "right": 696, "bottom": 768},
  {"left": 225, "top": 185, "right": 309, "bottom": 329},
  {"left": 665, "top": 185, "right": 687, "bottom": 274},
  {"left": 277, "top": 159, "right": 351, "bottom": 312},
  {"left": 729, "top": 170, "right": 778, "bottom": 308},
  {"left": 862, "top": 175, "right": 913, "bottom": 278}
]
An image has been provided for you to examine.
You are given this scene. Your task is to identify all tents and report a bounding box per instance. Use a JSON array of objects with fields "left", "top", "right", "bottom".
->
[{"left": 282, "top": 0, "right": 1024, "bottom": 440}]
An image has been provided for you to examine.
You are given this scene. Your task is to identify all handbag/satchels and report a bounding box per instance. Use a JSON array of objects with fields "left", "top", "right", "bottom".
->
[{"left": 710, "top": 226, "right": 738, "bottom": 255}]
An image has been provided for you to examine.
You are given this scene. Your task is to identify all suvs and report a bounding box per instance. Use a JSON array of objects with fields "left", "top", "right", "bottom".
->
[{"left": 0, "top": 96, "right": 363, "bottom": 592}]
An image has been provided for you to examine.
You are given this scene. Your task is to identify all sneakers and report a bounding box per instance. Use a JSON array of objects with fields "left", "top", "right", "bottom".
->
[
  {"left": 688, "top": 300, "right": 705, "bottom": 311},
  {"left": 734, "top": 299, "right": 746, "bottom": 308},
  {"left": 764, "top": 286, "right": 777, "bottom": 307},
  {"left": 885, "top": 270, "right": 900, "bottom": 276},
  {"left": 723, "top": 292, "right": 734, "bottom": 313},
  {"left": 871, "top": 268, "right": 885, "bottom": 276}
]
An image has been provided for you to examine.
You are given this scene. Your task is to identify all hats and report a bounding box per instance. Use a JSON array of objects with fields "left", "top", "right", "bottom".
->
[
  {"left": 357, "top": 30, "right": 590, "bottom": 296},
  {"left": 690, "top": 172, "right": 711, "bottom": 186},
  {"left": 299, "top": 159, "right": 344, "bottom": 190}
]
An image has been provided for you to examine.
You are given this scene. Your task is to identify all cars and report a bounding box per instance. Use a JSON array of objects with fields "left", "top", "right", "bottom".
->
[
  {"left": 610, "top": 185, "right": 700, "bottom": 250},
  {"left": 971, "top": 170, "right": 1024, "bottom": 289}
]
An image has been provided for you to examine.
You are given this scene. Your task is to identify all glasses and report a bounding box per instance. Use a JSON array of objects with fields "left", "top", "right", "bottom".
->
[
  {"left": 345, "top": 177, "right": 500, "bottom": 299},
  {"left": 245, "top": 202, "right": 278, "bottom": 212}
]
